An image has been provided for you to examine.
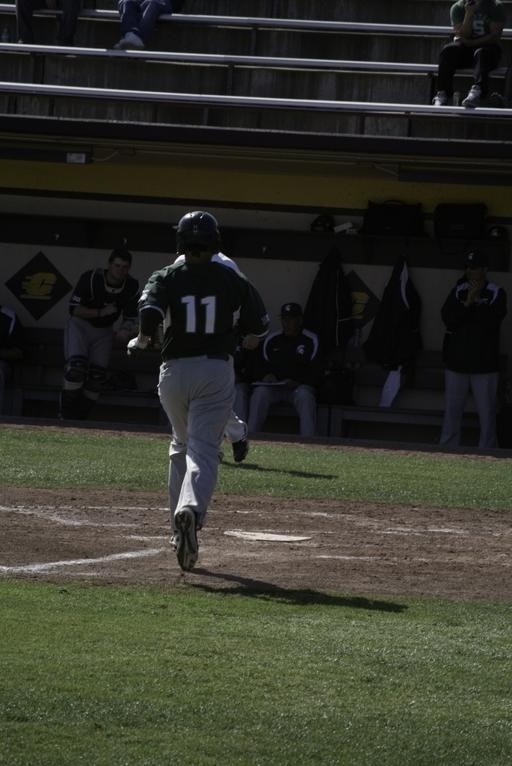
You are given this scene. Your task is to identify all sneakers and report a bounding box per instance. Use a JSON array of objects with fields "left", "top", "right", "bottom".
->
[
  {"left": 231, "top": 419, "right": 250, "bottom": 464},
  {"left": 433, "top": 78, "right": 485, "bottom": 107},
  {"left": 172, "top": 503, "right": 199, "bottom": 574},
  {"left": 113, "top": 30, "right": 146, "bottom": 49},
  {"left": 14, "top": 32, "right": 76, "bottom": 47}
]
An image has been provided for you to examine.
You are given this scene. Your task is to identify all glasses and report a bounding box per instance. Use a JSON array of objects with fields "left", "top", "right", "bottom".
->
[{"left": 463, "top": 262, "right": 488, "bottom": 272}]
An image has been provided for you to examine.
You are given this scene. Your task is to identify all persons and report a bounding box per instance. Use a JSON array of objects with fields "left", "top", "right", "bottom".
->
[
  {"left": 489, "top": 59, "right": 512, "bottom": 109},
  {"left": 166, "top": 241, "right": 251, "bottom": 465},
  {"left": 246, "top": 302, "right": 326, "bottom": 437},
  {"left": 15, "top": 0, "right": 100, "bottom": 61},
  {"left": 231, "top": 344, "right": 256, "bottom": 422},
  {"left": 0, "top": 306, "right": 27, "bottom": 390},
  {"left": 127, "top": 209, "right": 272, "bottom": 573},
  {"left": 433, "top": 0, "right": 503, "bottom": 106},
  {"left": 439, "top": 249, "right": 508, "bottom": 449},
  {"left": 58, "top": 246, "right": 142, "bottom": 421},
  {"left": 112, "top": 1, "right": 175, "bottom": 49}
]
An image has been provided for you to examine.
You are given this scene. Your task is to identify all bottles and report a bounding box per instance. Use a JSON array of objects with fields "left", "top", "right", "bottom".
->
[{"left": 453, "top": 92, "right": 461, "bottom": 105}]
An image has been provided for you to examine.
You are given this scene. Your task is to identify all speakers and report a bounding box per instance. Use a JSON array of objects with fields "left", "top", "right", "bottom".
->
[
  {"left": 366, "top": 200, "right": 423, "bottom": 235},
  {"left": 433, "top": 203, "right": 487, "bottom": 237}
]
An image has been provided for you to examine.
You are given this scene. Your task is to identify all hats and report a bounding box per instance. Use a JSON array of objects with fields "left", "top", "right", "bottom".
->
[
  {"left": 461, "top": 248, "right": 491, "bottom": 269},
  {"left": 276, "top": 301, "right": 307, "bottom": 319}
]
